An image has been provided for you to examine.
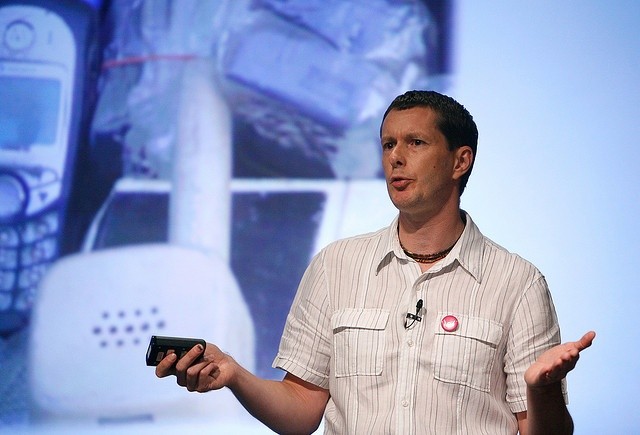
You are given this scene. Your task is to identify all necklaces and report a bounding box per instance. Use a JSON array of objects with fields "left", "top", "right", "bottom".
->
[{"left": 394, "top": 242, "right": 456, "bottom": 264}]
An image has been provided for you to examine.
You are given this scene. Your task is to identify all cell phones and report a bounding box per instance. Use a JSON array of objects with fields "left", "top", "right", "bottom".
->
[{"left": 0, "top": 4, "right": 96, "bottom": 335}]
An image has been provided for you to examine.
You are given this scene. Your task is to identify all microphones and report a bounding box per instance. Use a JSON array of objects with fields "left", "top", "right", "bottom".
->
[{"left": 405, "top": 297, "right": 425, "bottom": 329}]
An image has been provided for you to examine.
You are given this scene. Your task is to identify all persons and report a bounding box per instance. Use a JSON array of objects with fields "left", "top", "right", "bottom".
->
[{"left": 155, "top": 91, "right": 596, "bottom": 435}]
